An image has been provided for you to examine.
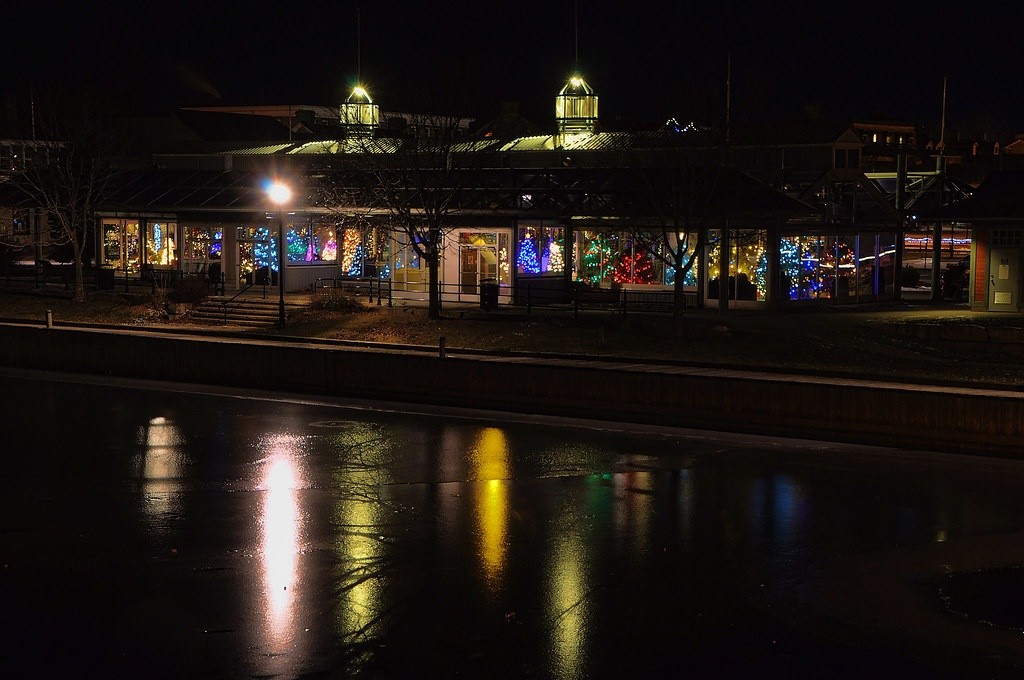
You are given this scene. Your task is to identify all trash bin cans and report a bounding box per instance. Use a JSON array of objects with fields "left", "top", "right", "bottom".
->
[
  {"left": 96, "top": 263, "right": 115, "bottom": 290},
  {"left": 479, "top": 277, "right": 500, "bottom": 311}
]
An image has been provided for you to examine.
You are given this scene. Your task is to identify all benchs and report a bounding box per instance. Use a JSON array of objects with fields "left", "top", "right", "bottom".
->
[
  {"left": 525, "top": 284, "right": 686, "bottom": 327},
  {"left": 341, "top": 281, "right": 389, "bottom": 299}
]
